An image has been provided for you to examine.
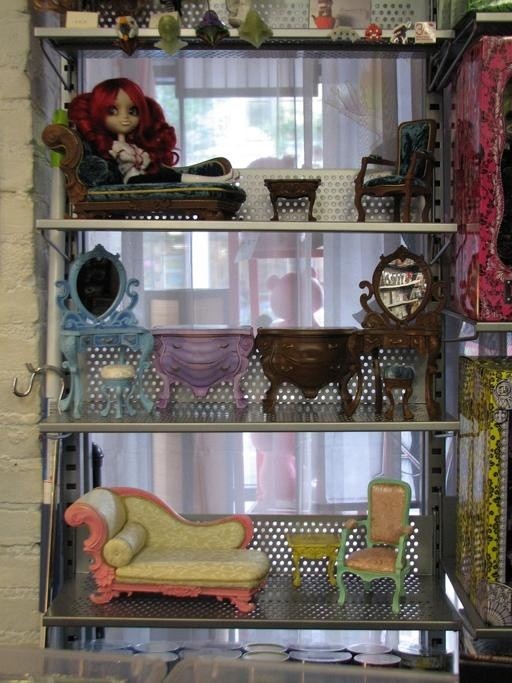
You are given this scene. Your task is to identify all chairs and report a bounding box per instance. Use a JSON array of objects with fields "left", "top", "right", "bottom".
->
[
  {"left": 43, "top": 123, "right": 245, "bottom": 221},
  {"left": 63, "top": 486, "right": 271, "bottom": 613},
  {"left": 354, "top": 119, "right": 438, "bottom": 222},
  {"left": 336, "top": 478, "right": 415, "bottom": 614}
]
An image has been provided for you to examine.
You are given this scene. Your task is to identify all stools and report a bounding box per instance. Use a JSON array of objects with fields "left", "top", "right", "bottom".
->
[
  {"left": 381, "top": 367, "right": 415, "bottom": 421},
  {"left": 98, "top": 365, "right": 137, "bottom": 419}
]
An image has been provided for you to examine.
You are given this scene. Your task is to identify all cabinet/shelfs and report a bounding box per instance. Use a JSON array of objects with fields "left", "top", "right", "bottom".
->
[
  {"left": 33, "top": 26, "right": 461, "bottom": 674},
  {"left": 428, "top": 7, "right": 512, "bottom": 642},
  {"left": 379, "top": 280, "right": 420, "bottom": 309}
]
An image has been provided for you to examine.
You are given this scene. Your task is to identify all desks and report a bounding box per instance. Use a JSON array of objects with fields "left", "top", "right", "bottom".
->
[
  {"left": 254, "top": 328, "right": 358, "bottom": 413},
  {"left": 59, "top": 328, "right": 155, "bottom": 421},
  {"left": 150, "top": 330, "right": 254, "bottom": 410},
  {"left": 346, "top": 328, "right": 441, "bottom": 416}
]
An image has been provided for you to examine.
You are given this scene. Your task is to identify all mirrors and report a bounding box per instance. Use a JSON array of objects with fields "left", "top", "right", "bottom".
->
[
  {"left": 359, "top": 246, "right": 449, "bottom": 327},
  {"left": 55, "top": 244, "right": 140, "bottom": 328}
]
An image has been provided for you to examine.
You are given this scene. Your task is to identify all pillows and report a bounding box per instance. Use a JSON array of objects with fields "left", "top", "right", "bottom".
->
[
  {"left": 75, "top": 156, "right": 123, "bottom": 187},
  {"left": 102, "top": 522, "right": 148, "bottom": 567}
]
